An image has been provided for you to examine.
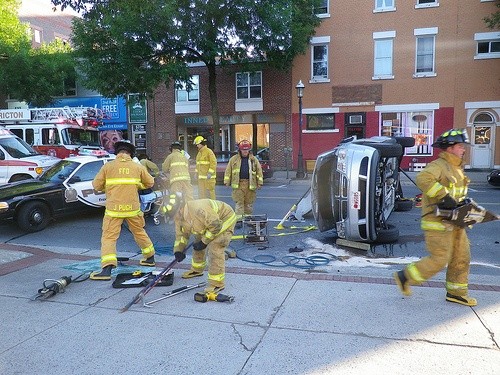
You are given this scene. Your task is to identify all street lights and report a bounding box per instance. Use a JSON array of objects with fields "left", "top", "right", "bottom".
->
[{"left": 294, "top": 80, "right": 305, "bottom": 178}]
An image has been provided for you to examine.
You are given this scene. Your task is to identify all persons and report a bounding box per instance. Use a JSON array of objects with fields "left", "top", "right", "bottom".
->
[
  {"left": 158, "top": 191, "right": 237, "bottom": 294},
  {"left": 193, "top": 136, "right": 217, "bottom": 200},
  {"left": 139, "top": 153, "right": 160, "bottom": 177},
  {"left": 224, "top": 140, "right": 263, "bottom": 214},
  {"left": 89, "top": 139, "right": 155, "bottom": 280},
  {"left": 394, "top": 127, "right": 477, "bottom": 306},
  {"left": 162, "top": 141, "right": 194, "bottom": 225},
  {"left": 100, "top": 130, "right": 123, "bottom": 151}
]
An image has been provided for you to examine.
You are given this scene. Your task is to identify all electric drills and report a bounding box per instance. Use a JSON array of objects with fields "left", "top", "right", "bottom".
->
[{"left": 194, "top": 291, "right": 234, "bottom": 303}]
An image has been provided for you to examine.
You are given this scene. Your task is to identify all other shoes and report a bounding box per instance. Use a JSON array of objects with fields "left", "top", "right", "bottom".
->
[
  {"left": 203, "top": 284, "right": 225, "bottom": 292},
  {"left": 89, "top": 266, "right": 112, "bottom": 280},
  {"left": 445, "top": 292, "right": 478, "bottom": 305},
  {"left": 181, "top": 270, "right": 203, "bottom": 278},
  {"left": 393, "top": 269, "right": 412, "bottom": 296},
  {"left": 140, "top": 257, "right": 156, "bottom": 267}
]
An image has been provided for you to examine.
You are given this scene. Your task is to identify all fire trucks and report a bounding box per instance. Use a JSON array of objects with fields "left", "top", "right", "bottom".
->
[{"left": 0, "top": 103, "right": 110, "bottom": 160}]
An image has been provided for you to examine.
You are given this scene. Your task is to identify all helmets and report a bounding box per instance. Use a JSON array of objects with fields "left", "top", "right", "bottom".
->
[
  {"left": 115, "top": 139, "right": 136, "bottom": 154},
  {"left": 169, "top": 140, "right": 183, "bottom": 151},
  {"left": 162, "top": 194, "right": 180, "bottom": 218},
  {"left": 193, "top": 136, "right": 204, "bottom": 146},
  {"left": 430, "top": 128, "right": 471, "bottom": 149}
]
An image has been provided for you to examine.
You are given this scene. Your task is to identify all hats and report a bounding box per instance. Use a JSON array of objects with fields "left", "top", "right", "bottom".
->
[{"left": 237, "top": 140, "right": 251, "bottom": 150}]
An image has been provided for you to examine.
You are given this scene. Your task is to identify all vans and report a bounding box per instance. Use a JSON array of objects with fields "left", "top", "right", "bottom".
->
[{"left": 0, "top": 125, "right": 62, "bottom": 185}]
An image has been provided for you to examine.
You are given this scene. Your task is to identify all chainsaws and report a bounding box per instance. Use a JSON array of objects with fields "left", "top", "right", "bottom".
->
[{"left": 30, "top": 275, "right": 72, "bottom": 300}]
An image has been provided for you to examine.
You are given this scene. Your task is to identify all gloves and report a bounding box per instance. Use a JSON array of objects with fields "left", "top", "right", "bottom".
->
[
  {"left": 193, "top": 241, "right": 207, "bottom": 250},
  {"left": 441, "top": 196, "right": 457, "bottom": 208},
  {"left": 174, "top": 251, "right": 187, "bottom": 263}
]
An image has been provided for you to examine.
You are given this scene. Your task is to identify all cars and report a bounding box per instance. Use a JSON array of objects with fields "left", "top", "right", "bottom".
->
[
  {"left": 0, "top": 150, "right": 169, "bottom": 233},
  {"left": 486, "top": 170, "right": 500, "bottom": 186},
  {"left": 311, "top": 134, "right": 415, "bottom": 251},
  {"left": 189, "top": 150, "right": 273, "bottom": 186}
]
